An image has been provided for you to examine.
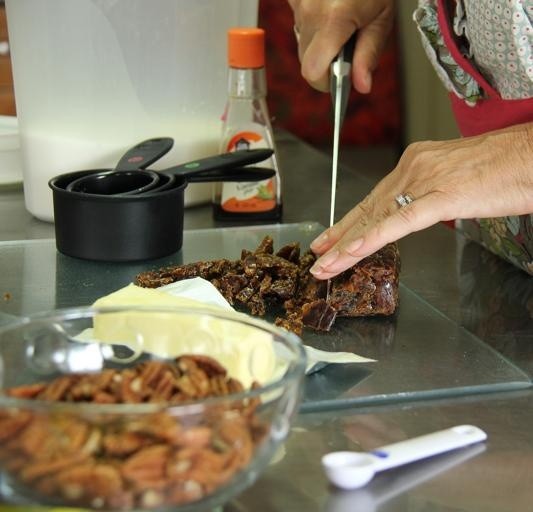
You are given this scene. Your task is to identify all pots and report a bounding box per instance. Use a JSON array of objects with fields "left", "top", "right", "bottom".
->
[{"left": 44, "top": 133, "right": 280, "bottom": 268}]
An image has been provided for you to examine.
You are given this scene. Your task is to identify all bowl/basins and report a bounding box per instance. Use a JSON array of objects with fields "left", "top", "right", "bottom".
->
[{"left": 2, "top": 304, "right": 307, "bottom": 512}]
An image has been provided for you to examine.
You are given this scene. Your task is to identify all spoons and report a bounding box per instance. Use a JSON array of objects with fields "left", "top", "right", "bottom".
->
[
  {"left": 318, "top": 419, "right": 488, "bottom": 489},
  {"left": 320, "top": 441, "right": 486, "bottom": 511}
]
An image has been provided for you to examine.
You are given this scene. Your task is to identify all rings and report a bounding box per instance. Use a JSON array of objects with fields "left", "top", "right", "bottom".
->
[
  {"left": 294, "top": 25, "right": 301, "bottom": 42},
  {"left": 394, "top": 193, "right": 406, "bottom": 207},
  {"left": 404, "top": 195, "right": 412, "bottom": 203}
]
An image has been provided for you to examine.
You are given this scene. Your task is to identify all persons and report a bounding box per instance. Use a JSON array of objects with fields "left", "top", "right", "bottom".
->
[{"left": 289, "top": 1, "right": 533, "bottom": 283}]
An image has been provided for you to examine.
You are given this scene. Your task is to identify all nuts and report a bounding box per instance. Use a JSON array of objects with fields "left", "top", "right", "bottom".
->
[{"left": 1, "top": 354, "right": 267, "bottom": 508}]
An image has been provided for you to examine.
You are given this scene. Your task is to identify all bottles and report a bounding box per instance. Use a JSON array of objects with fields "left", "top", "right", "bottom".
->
[{"left": 214, "top": 27, "right": 289, "bottom": 224}]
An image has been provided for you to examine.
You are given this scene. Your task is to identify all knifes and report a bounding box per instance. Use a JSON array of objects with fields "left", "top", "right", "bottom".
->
[{"left": 321, "top": 31, "right": 365, "bottom": 302}]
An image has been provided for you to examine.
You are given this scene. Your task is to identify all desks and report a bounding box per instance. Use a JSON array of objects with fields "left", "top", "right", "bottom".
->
[{"left": 0, "top": 120, "right": 532, "bottom": 511}]
